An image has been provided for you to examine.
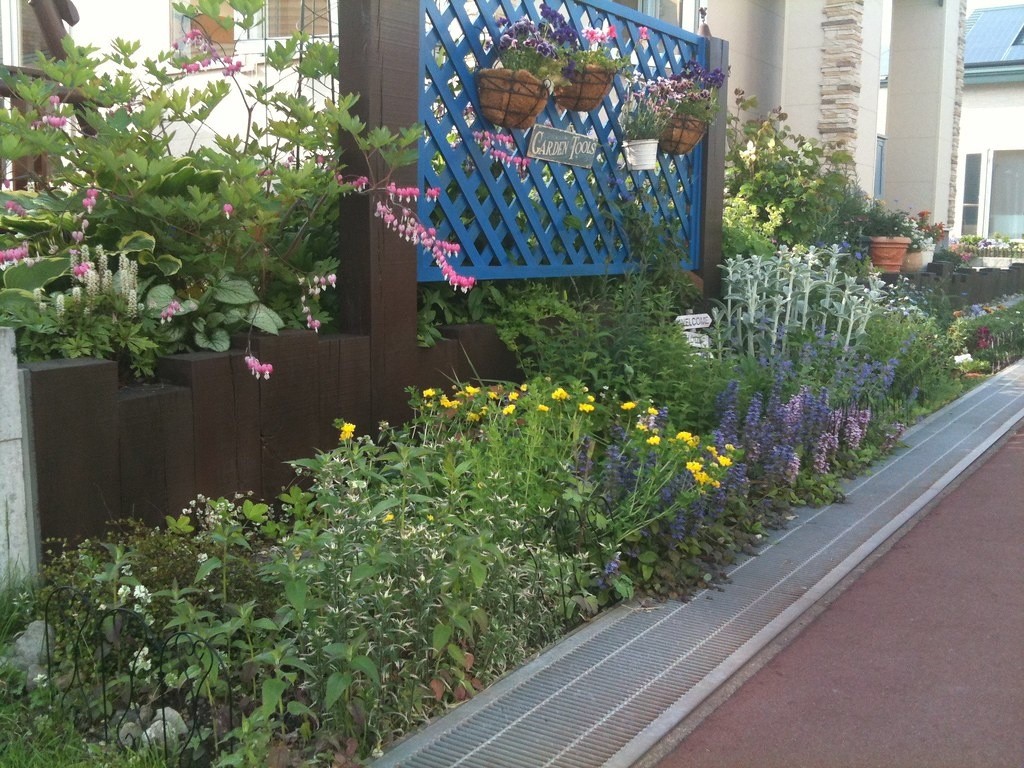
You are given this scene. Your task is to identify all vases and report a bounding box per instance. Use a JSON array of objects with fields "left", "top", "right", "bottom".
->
[
  {"left": 865, "top": 236, "right": 911, "bottom": 273},
  {"left": 900, "top": 243, "right": 935, "bottom": 272},
  {"left": 621, "top": 139, "right": 659, "bottom": 170}
]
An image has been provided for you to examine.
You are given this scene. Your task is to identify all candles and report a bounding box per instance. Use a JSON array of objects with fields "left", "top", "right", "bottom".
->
[{"left": 700, "top": 0, "right": 707, "bottom": 9}]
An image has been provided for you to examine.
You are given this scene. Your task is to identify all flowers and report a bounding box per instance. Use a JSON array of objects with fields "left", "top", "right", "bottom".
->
[
  {"left": 492, "top": 15, "right": 576, "bottom": 96},
  {"left": 863, "top": 202, "right": 936, "bottom": 253},
  {"left": 622, "top": 71, "right": 710, "bottom": 140},
  {"left": 667, "top": 59, "right": 724, "bottom": 126},
  {"left": 574, "top": 18, "right": 650, "bottom": 80},
  {"left": 910, "top": 210, "right": 945, "bottom": 243}
]
